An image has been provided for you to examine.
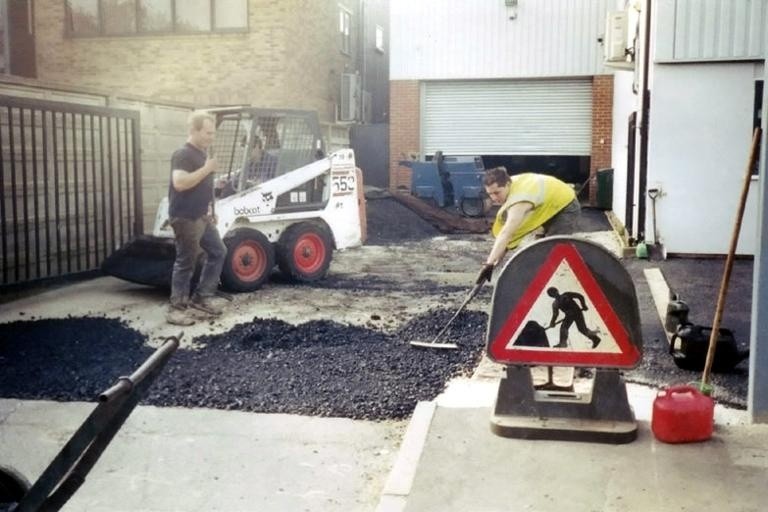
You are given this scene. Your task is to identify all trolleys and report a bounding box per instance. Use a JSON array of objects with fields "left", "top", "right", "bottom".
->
[{"left": 0, "top": 329, "right": 185, "bottom": 512}]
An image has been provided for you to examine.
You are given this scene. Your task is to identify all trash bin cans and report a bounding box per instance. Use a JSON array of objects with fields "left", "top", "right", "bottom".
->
[{"left": 595, "top": 168, "right": 614, "bottom": 209}]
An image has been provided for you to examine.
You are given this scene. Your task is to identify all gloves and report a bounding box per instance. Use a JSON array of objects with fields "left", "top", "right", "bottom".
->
[{"left": 475, "top": 261, "right": 494, "bottom": 286}]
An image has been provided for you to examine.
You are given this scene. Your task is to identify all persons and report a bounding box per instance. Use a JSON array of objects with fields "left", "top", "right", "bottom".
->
[
  {"left": 474, "top": 166, "right": 581, "bottom": 288},
  {"left": 164, "top": 109, "right": 229, "bottom": 327},
  {"left": 216, "top": 134, "right": 278, "bottom": 197}
]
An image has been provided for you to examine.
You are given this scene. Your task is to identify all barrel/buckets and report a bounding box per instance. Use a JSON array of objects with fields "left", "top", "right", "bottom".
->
[{"left": 651, "top": 385, "right": 715, "bottom": 445}]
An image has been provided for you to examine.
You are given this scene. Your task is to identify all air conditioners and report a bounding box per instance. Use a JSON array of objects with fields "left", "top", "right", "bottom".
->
[
  {"left": 361, "top": 89, "right": 373, "bottom": 122},
  {"left": 606, "top": 11, "right": 629, "bottom": 62},
  {"left": 340, "top": 73, "right": 361, "bottom": 121}
]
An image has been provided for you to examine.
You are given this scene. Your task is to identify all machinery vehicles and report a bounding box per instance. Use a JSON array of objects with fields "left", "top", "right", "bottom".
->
[{"left": 101, "top": 105, "right": 366, "bottom": 292}]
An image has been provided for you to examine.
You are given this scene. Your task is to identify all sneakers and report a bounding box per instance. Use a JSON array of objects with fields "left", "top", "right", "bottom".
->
[{"left": 166, "top": 298, "right": 222, "bottom": 326}]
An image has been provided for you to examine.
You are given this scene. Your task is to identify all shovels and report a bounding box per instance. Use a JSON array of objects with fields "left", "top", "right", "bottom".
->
[{"left": 646, "top": 188, "right": 667, "bottom": 263}]
